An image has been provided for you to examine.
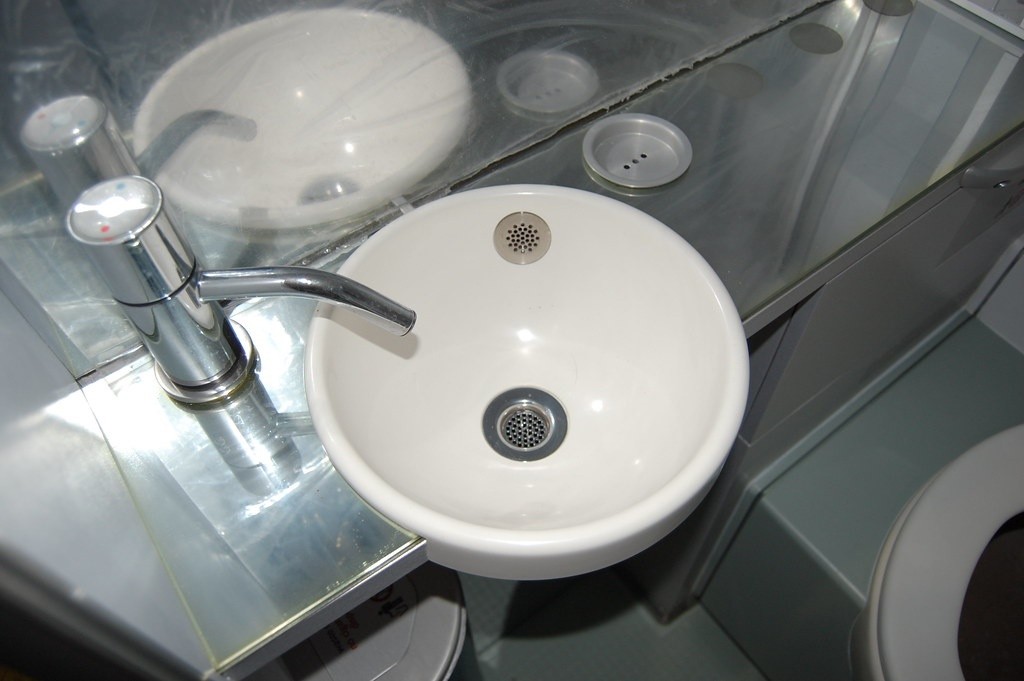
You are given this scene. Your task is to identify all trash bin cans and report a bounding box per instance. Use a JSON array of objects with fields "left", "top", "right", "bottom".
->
[{"left": 279, "top": 561, "right": 483, "bottom": 681}]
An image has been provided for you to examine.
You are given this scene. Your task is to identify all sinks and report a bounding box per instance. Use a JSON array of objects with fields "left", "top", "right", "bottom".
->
[
  {"left": 131, "top": 5, "right": 474, "bottom": 235},
  {"left": 302, "top": 180, "right": 752, "bottom": 583}
]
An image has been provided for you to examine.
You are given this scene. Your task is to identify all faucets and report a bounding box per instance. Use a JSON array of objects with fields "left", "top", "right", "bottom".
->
[
  {"left": 194, "top": 373, "right": 316, "bottom": 497},
  {"left": 19, "top": 94, "right": 258, "bottom": 207},
  {"left": 65, "top": 174, "right": 418, "bottom": 410}
]
